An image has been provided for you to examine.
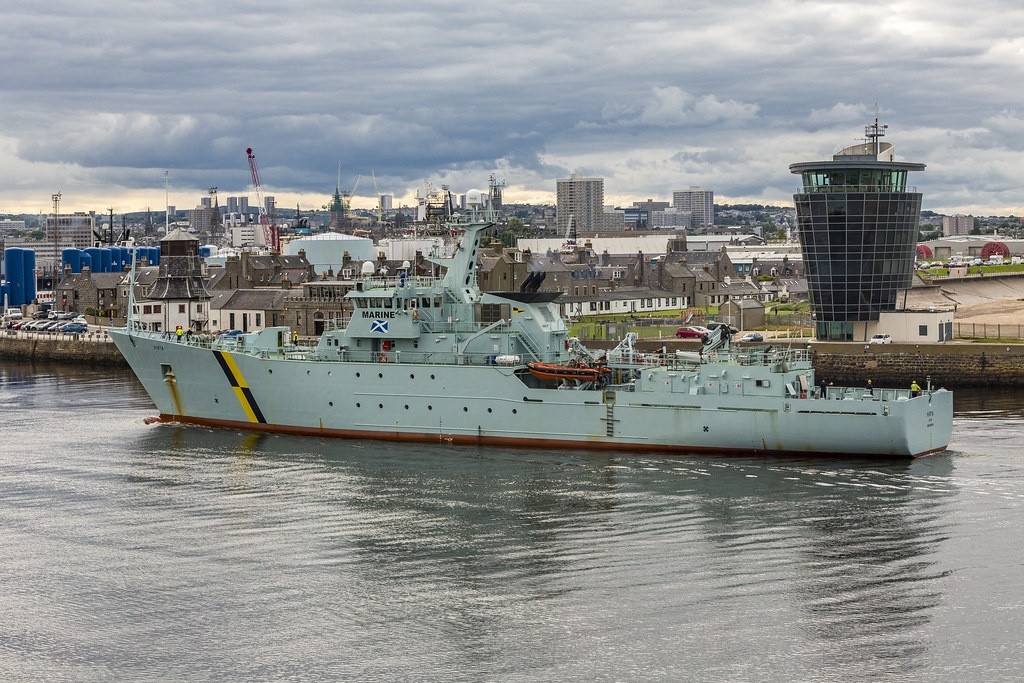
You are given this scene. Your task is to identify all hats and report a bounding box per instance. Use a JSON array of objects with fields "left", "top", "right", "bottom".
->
[{"left": 912, "top": 381, "right": 916, "bottom": 383}]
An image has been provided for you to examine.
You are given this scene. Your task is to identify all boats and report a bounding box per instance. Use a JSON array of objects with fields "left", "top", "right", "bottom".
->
[{"left": 525, "top": 360, "right": 610, "bottom": 387}]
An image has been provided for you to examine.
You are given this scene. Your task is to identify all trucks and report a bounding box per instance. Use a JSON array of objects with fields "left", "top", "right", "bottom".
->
[{"left": 6, "top": 308, "right": 23, "bottom": 320}]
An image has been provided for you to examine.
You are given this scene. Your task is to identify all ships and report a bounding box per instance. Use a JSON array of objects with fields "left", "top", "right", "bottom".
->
[{"left": 100, "top": 220, "right": 957, "bottom": 461}]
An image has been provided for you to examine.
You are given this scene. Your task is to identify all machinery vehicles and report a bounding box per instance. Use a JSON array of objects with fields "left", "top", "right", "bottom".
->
[
  {"left": 245, "top": 146, "right": 284, "bottom": 254},
  {"left": 328, "top": 156, "right": 386, "bottom": 222}
]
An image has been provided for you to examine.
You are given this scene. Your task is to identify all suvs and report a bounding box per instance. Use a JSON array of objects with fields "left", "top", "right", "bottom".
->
[{"left": 707, "top": 323, "right": 740, "bottom": 333}]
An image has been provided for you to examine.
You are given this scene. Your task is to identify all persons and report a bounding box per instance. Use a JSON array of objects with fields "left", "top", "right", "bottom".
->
[
  {"left": 187, "top": 328, "right": 193, "bottom": 341},
  {"left": 175, "top": 326, "right": 183, "bottom": 343},
  {"left": 399, "top": 270, "right": 408, "bottom": 287},
  {"left": 656, "top": 345, "right": 663, "bottom": 358},
  {"left": 574, "top": 335, "right": 580, "bottom": 345},
  {"left": 866, "top": 380, "right": 874, "bottom": 395},
  {"left": 143, "top": 325, "right": 146, "bottom": 330},
  {"left": 819, "top": 380, "right": 828, "bottom": 399},
  {"left": 910, "top": 381, "right": 921, "bottom": 397},
  {"left": 293, "top": 331, "right": 299, "bottom": 346}
]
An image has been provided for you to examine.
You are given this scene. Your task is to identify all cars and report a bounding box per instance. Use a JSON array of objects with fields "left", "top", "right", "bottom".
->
[
  {"left": 2, "top": 321, "right": 18, "bottom": 329},
  {"left": 739, "top": 333, "right": 763, "bottom": 343},
  {"left": 692, "top": 326, "right": 713, "bottom": 334},
  {"left": 30, "top": 321, "right": 50, "bottom": 330},
  {"left": 63, "top": 312, "right": 78, "bottom": 319},
  {"left": 59, "top": 322, "right": 89, "bottom": 331},
  {"left": 869, "top": 332, "right": 893, "bottom": 345},
  {"left": 33, "top": 310, "right": 48, "bottom": 319},
  {"left": 71, "top": 318, "right": 87, "bottom": 326},
  {"left": 38, "top": 322, "right": 57, "bottom": 331},
  {"left": 11, "top": 321, "right": 29, "bottom": 330},
  {"left": 914, "top": 254, "right": 1024, "bottom": 270},
  {"left": 47, "top": 311, "right": 66, "bottom": 320},
  {"left": 20, "top": 321, "right": 36, "bottom": 330},
  {"left": 675, "top": 327, "right": 707, "bottom": 339},
  {"left": 47, "top": 322, "right": 68, "bottom": 331},
  {"left": 921, "top": 264, "right": 930, "bottom": 270}
]
